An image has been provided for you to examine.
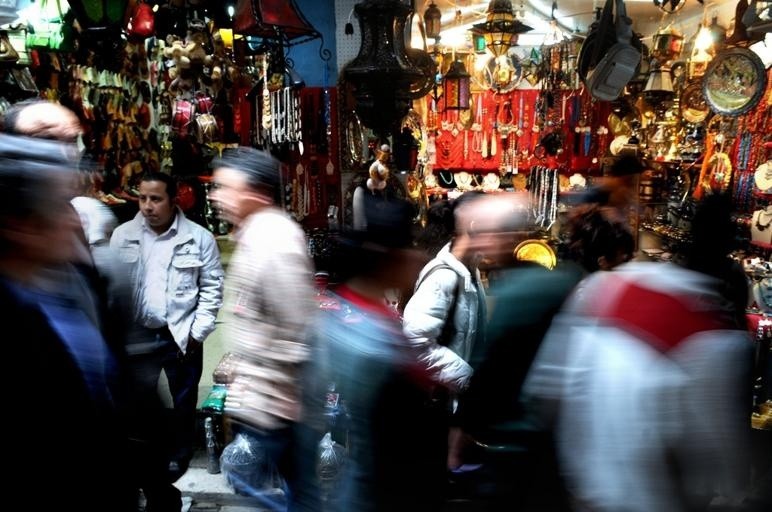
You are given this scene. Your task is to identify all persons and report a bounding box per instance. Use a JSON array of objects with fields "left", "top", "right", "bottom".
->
[{"left": 0, "top": 94, "right": 771, "bottom": 512}]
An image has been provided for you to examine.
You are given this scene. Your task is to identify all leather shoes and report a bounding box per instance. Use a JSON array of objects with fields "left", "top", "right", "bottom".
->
[
  {"left": 65, "top": 64, "right": 146, "bottom": 206},
  {"left": 30, "top": 48, "right": 64, "bottom": 103}
]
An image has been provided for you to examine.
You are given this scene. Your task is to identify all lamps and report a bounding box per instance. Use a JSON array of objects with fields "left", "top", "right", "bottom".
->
[
  {"left": 474, "top": 0, "right": 534, "bottom": 58},
  {"left": 439, "top": 59, "right": 471, "bottom": 111},
  {"left": 423, "top": 0, "right": 442, "bottom": 38}
]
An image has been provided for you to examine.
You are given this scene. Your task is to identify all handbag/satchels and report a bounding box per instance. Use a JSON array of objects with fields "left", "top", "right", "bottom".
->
[{"left": 574, "top": 0, "right": 642, "bottom": 101}]
